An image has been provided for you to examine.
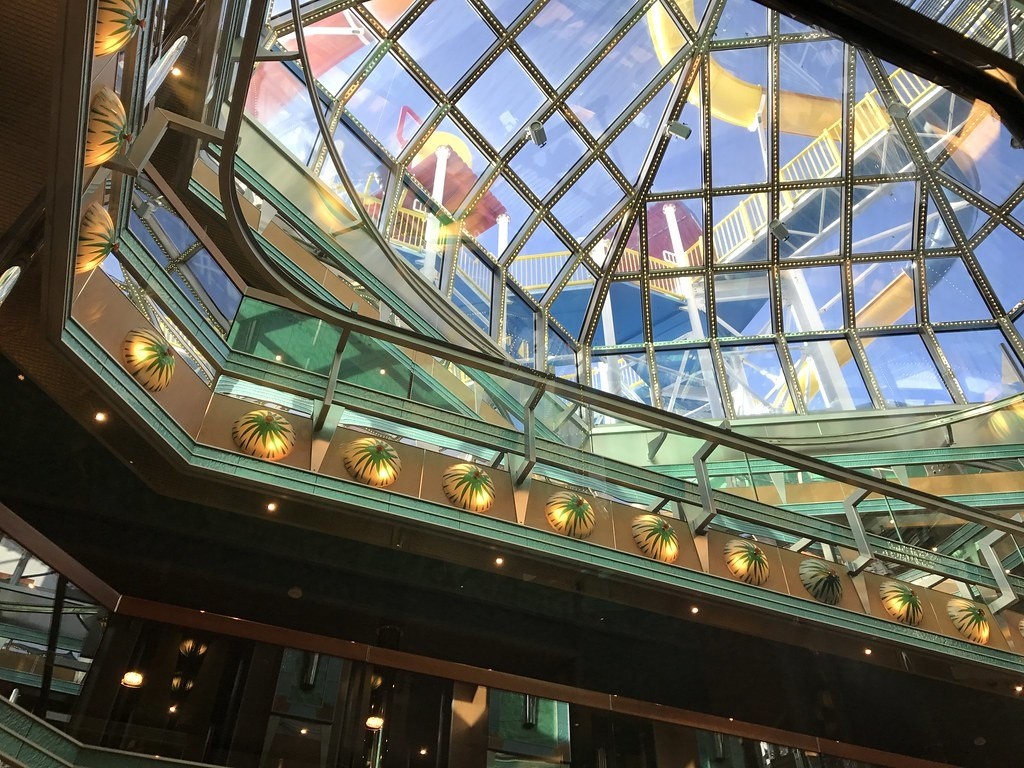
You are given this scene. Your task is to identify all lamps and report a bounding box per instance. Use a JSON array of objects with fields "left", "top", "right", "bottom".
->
[
  {"left": 725, "top": 539, "right": 770, "bottom": 586},
  {"left": 631, "top": 515, "right": 679, "bottom": 562},
  {"left": 84, "top": 85, "right": 134, "bottom": 169},
  {"left": 947, "top": 598, "right": 990, "bottom": 645},
  {"left": 120, "top": 331, "right": 175, "bottom": 392},
  {"left": 94, "top": 0, "right": 146, "bottom": 58},
  {"left": 179, "top": 634, "right": 207, "bottom": 658},
  {"left": 442, "top": 464, "right": 495, "bottom": 512},
  {"left": 879, "top": 580, "right": 924, "bottom": 627},
  {"left": 343, "top": 438, "right": 401, "bottom": 487},
  {"left": 799, "top": 559, "right": 842, "bottom": 605},
  {"left": 76, "top": 202, "right": 119, "bottom": 274},
  {"left": 170, "top": 663, "right": 203, "bottom": 700},
  {"left": 232, "top": 410, "right": 295, "bottom": 461},
  {"left": 365, "top": 695, "right": 384, "bottom": 730},
  {"left": 544, "top": 491, "right": 595, "bottom": 539}
]
